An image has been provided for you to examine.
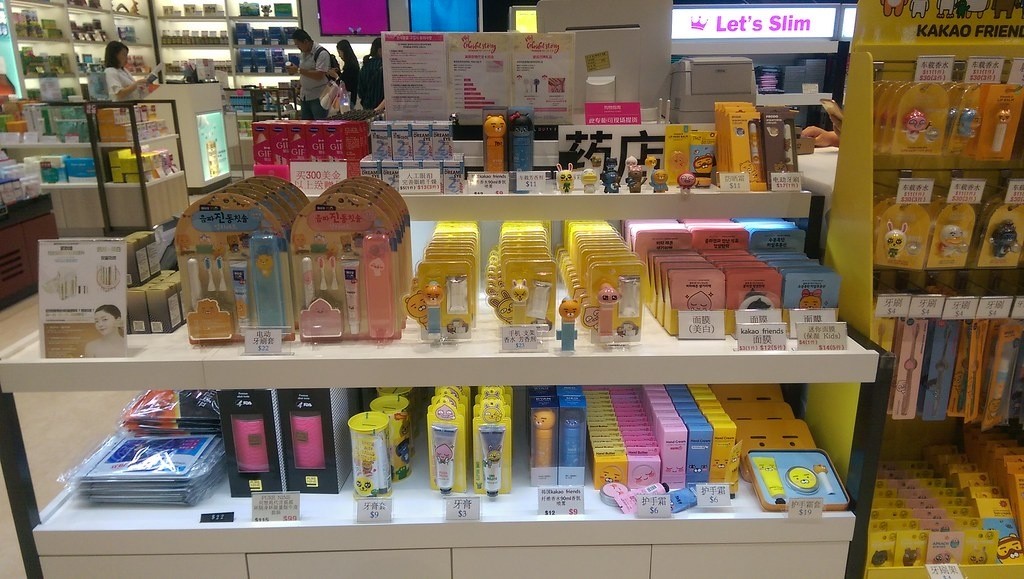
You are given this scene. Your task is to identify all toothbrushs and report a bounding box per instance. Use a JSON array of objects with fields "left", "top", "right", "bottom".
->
[
  {"left": 216, "top": 256, "right": 229, "bottom": 291},
  {"left": 316, "top": 255, "right": 328, "bottom": 291},
  {"left": 202, "top": 256, "right": 215, "bottom": 292},
  {"left": 870, "top": 316, "right": 1023, "bottom": 432},
  {"left": 328, "top": 256, "right": 340, "bottom": 291}
]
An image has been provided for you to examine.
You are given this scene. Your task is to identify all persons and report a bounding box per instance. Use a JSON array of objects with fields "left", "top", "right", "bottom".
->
[
  {"left": 284, "top": 29, "right": 331, "bottom": 120},
  {"left": 802, "top": 113, "right": 842, "bottom": 146},
  {"left": 104, "top": 42, "right": 147, "bottom": 102},
  {"left": 85, "top": 305, "right": 125, "bottom": 357},
  {"left": 327, "top": 39, "right": 360, "bottom": 111},
  {"left": 358, "top": 38, "right": 385, "bottom": 111}
]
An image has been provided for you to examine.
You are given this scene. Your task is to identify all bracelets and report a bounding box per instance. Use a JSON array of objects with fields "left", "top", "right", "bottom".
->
[
  {"left": 336, "top": 76, "right": 339, "bottom": 80},
  {"left": 298, "top": 67, "right": 300, "bottom": 74}
]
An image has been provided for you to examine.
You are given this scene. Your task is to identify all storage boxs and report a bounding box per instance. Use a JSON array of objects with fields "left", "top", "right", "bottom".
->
[
  {"left": 98, "top": 104, "right": 169, "bottom": 140},
  {"left": 130, "top": 230, "right": 161, "bottom": 278},
  {"left": 126, "top": 54, "right": 148, "bottom": 73},
  {"left": 804, "top": 70, "right": 825, "bottom": 80},
  {"left": 99, "top": 31, "right": 109, "bottom": 41},
  {"left": 776, "top": 80, "right": 802, "bottom": 94},
  {"left": 778, "top": 66, "right": 806, "bottom": 73},
  {"left": 119, "top": 236, "right": 151, "bottom": 287},
  {"left": 863, "top": 423, "right": 1024, "bottom": 567},
  {"left": 795, "top": 59, "right": 827, "bottom": 70},
  {"left": 583, "top": 384, "right": 854, "bottom": 514},
  {"left": 90, "top": 63, "right": 103, "bottom": 72},
  {"left": 230, "top": 79, "right": 467, "bottom": 195},
  {"left": 93, "top": 19, "right": 103, "bottom": 29},
  {"left": 0, "top": 149, "right": 97, "bottom": 206},
  {"left": 108, "top": 144, "right": 173, "bottom": 183},
  {"left": 161, "top": 2, "right": 229, "bottom": 46},
  {"left": 235, "top": 1, "right": 300, "bottom": 74},
  {"left": 0, "top": 11, "right": 96, "bottom": 143},
  {"left": 779, "top": 72, "right": 805, "bottom": 81},
  {"left": 128, "top": 269, "right": 187, "bottom": 335}
]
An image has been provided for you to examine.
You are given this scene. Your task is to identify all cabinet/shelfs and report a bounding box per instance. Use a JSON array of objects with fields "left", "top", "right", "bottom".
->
[
  {"left": 150, "top": 0, "right": 304, "bottom": 170},
  {"left": 785, "top": 0, "right": 1024, "bottom": 579},
  {"left": 225, "top": 88, "right": 296, "bottom": 141},
  {"left": 0, "top": 192, "right": 897, "bottom": 579},
  {"left": 670, "top": 38, "right": 851, "bottom": 258},
  {"left": 0, "top": 0, "right": 162, "bottom": 168}
]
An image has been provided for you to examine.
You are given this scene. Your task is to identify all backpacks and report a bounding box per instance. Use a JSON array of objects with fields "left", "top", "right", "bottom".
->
[{"left": 314, "top": 47, "right": 340, "bottom": 84}]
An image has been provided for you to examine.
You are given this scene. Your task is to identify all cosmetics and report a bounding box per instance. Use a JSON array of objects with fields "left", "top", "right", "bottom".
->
[
  {"left": 123, "top": 230, "right": 186, "bottom": 336},
  {"left": 583, "top": 384, "right": 742, "bottom": 517},
  {"left": 0, "top": 101, "right": 179, "bottom": 203},
  {"left": 206, "top": 140, "right": 219, "bottom": 178},
  {"left": 873, "top": 79, "right": 1024, "bottom": 270}
]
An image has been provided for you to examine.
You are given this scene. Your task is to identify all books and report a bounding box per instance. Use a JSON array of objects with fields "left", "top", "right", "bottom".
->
[{"left": 137, "top": 63, "right": 164, "bottom": 92}]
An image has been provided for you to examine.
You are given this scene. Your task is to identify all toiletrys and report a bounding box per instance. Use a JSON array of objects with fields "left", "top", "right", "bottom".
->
[
  {"left": 430, "top": 424, "right": 458, "bottom": 497},
  {"left": 347, "top": 387, "right": 415, "bottom": 502},
  {"left": 341, "top": 260, "right": 361, "bottom": 334},
  {"left": 230, "top": 260, "right": 249, "bottom": 336},
  {"left": 477, "top": 424, "right": 507, "bottom": 498}
]
